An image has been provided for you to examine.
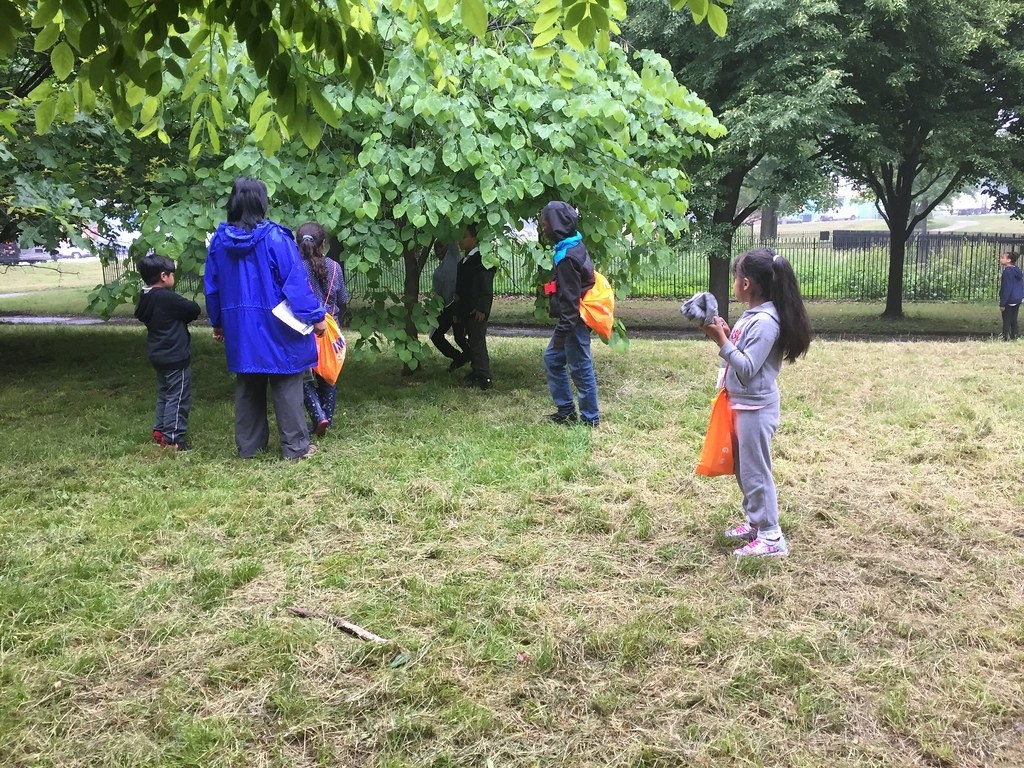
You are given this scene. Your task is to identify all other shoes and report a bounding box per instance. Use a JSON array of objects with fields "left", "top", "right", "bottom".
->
[
  {"left": 447, "top": 360, "right": 470, "bottom": 373},
  {"left": 543, "top": 405, "right": 578, "bottom": 426},
  {"left": 293, "top": 445, "right": 317, "bottom": 462},
  {"left": 465, "top": 376, "right": 479, "bottom": 388},
  {"left": 153, "top": 431, "right": 163, "bottom": 446},
  {"left": 162, "top": 435, "right": 193, "bottom": 453},
  {"left": 465, "top": 378, "right": 493, "bottom": 391},
  {"left": 580, "top": 415, "right": 599, "bottom": 427},
  {"left": 463, "top": 373, "right": 473, "bottom": 380}
]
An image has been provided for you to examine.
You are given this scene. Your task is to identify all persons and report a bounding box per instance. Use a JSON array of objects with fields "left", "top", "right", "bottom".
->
[
  {"left": 429, "top": 237, "right": 472, "bottom": 372},
  {"left": 999, "top": 251, "right": 1024, "bottom": 342},
  {"left": 454, "top": 222, "right": 495, "bottom": 391},
  {"left": 203, "top": 179, "right": 327, "bottom": 463},
  {"left": 695, "top": 247, "right": 809, "bottom": 558},
  {"left": 134, "top": 255, "right": 201, "bottom": 450},
  {"left": 541, "top": 200, "right": 600, "bottom": 428},
  {"left": 296, "top": 222, "right": 349, "bottom": 435}
]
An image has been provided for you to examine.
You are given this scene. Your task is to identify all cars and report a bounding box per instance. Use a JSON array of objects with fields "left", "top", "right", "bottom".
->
[{"left": 55, "top": 242, "right": 91, "bottom": 259}]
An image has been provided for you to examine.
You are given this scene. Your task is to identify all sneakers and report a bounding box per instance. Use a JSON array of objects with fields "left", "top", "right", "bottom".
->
[
  {"left": 725, "top": 524, "right": 757, "bottom": 540},
  {"left": 735, "top": 536, "right": 789, "bottom": 559}
]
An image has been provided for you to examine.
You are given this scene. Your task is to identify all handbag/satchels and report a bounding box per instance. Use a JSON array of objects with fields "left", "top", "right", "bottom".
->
[
  {"left": 696, "top": 388, "right": 738, "bottom": 478},
  {"left": 579, "top": 270, "right": 615, "bottom": 339},
  {"left": 313, "top": 312, "right": 346, "bottom": 387}
]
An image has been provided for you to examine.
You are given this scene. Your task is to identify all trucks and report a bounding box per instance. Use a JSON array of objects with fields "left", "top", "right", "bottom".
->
[{"left": 1, "top": 236, "right": 51, "bottom": 267}]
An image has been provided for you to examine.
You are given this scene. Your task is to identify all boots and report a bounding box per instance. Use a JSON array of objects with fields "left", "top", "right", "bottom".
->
[
  {"left": 308, "top": 384, "right": 337, "bottom": 433},
  {"left": 303, "top": 382, "right": 328, "bottom": 437}
]
